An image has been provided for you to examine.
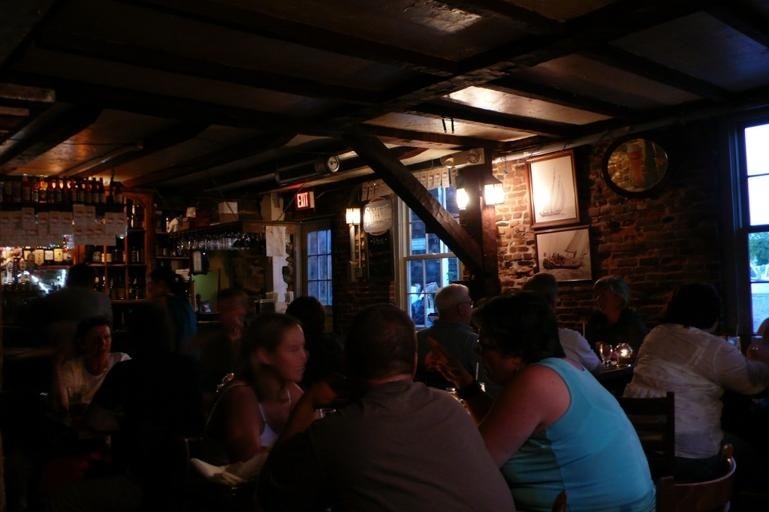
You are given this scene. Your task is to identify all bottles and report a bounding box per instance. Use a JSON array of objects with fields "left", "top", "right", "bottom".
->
[
  {"left": 3, "top": 174, "right": 106, "bottom": 205},
  {"left": 129, "top": 203, "right": 137, "bottom": 230},
  {"left": 90, "top": 275, "right": 143, "bottom": 299},
  {"left": 111, "top": 178, "right": 125, "bottom": 207},
  {"left": 1, "top": 245, "right": 63, "bottom": 265},
  {"left": 86, "top": 243, "right": 145, "bottom": 264},
  {"left": 175, "top": 233, "right": 254, "bottom": 255}
]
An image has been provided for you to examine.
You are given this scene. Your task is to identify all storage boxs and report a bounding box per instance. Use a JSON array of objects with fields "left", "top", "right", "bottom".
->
[
  {"left": 166, "top": 203, "right": 210, "bottom": 234},
  {"left": 217, "top": 201, "right": 239, "bottom": 213},
  {"left": 219, "top": 213, "right": 238, "bottom": 222}
]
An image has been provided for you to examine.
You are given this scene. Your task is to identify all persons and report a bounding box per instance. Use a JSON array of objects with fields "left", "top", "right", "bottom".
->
[
  {"left": 521, "top": 273, "right": 602, "bottom": 375},
  {"left": 620, "top": 278, "right": 769, "bottom": 482},
  {"left": 0, "top": 245, "right": 518, "bottom": 511},
  {"left": 467, "top": 286, "right": 658, "bottom": 511},
  {"left": 585, "top": 274, "right": 648, "bottom": 397}
]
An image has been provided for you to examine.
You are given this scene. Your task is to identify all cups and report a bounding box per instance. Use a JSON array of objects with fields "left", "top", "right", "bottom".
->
[{"left": 599, "top": 342, "right": 612, "bottom": 369}]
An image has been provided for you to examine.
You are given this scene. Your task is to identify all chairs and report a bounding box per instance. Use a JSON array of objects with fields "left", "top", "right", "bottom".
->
[
  {"left": 657, "top": 445, "right": 737, "bottom": 512},
  {"left": 614, "top": 392, "right": 676, "bottom": 483}
]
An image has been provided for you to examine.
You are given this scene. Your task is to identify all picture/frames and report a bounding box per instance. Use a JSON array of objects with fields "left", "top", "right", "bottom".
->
[
  {"left": 525, "top": 150, "right": 581, "bottom": 229},
  {"left": 533, "top": 225, "right": 595, "bottom": 286}
]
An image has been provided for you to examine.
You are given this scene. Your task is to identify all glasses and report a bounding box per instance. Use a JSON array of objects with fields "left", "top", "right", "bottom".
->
[{"left": 457, "top": 299, "right": 474, "bottom": 309}]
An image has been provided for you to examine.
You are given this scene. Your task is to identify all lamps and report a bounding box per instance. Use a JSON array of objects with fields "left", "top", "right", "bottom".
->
[
  {"left": 345, "top": 207, "right": 352, "bottom": 282},
  {"left": 481, "top": 171, "right": 508, "bottom": 209},
  {"left": 454, "top": 187, "right": 472, "bottom": 212},
  {"left": 70, "top": 207, "right": 76, "bottom": 226},
  {"left": 352, "top": 208, "right": 362, "bottom": 277}
]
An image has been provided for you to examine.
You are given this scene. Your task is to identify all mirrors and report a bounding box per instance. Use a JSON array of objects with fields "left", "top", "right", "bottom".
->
[{"left": 601, "top": 134, "right": 672, "bottom": 197}]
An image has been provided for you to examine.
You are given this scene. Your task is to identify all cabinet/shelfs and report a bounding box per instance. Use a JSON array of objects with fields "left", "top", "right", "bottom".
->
[
  {"left": 0, "top": 244, "right": 78, "bottom": 301},
  {"left": 245, "top": 290, "right": 273, "bottom": 316},
  {"left": 80, "top": 193, "right": 154, "bottom": 303}
]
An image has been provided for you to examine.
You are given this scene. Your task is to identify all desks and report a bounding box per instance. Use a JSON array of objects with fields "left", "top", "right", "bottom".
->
[{"left": 594, "top": 358, "right": 632, "bottom": 395}]
